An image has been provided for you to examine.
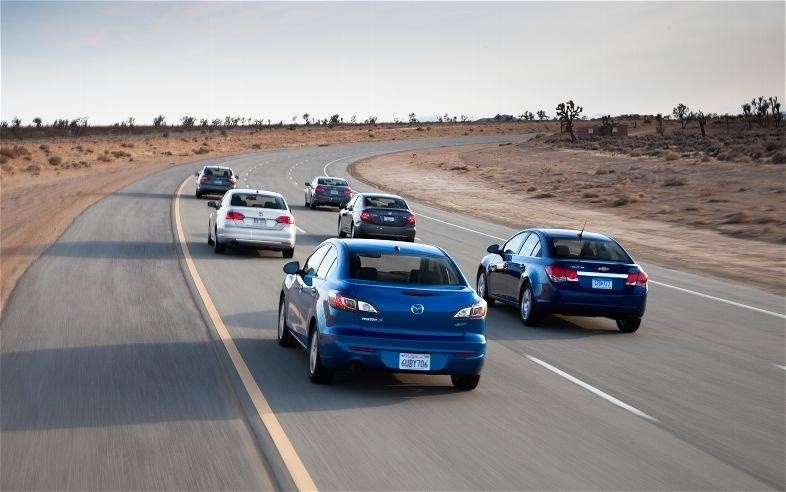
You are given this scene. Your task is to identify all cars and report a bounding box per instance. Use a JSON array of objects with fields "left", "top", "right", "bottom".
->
[
  {"left": 474, "top": 227, "right": 649, "bottom": 334},
  {"left": 273, "top": 235, "right": 491, "bottom": 394},
  {"left": 304, "top": 176, "right": 354, "bottom": 210},
  {"left": 337, "top": 192, "right": 417, "bottom": 242},
  {"left": 194, "top": 165, "right": 240, "bottom": 199},
  {"left": 206, "top": 187, "right": 298, "bottom": 259}
]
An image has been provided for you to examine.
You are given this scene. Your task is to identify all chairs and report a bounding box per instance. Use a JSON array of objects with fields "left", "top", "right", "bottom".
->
[
  {"left": 223, "top": 171, "right": 228, "bottom": 176},
  {"left": 234, "top": 200, "right": 277, "bottom": 208},
  {"left": 336, "top": 182, "right": 340, "bottom": 185},
  {"left": 206, "top": 170, "right": 212, "bottom": 175},
  {"left": 556, "top": 245, "right": 595, "bottom": 257},
  {"left": 366, "top": 200, "right": 399, "bottom": 208},
  {"left": 319, "top": 181, "right": 327, "bottom": 185},
  {"left": 350, "top": 254, "right": 440, "bottom": 283}
]
